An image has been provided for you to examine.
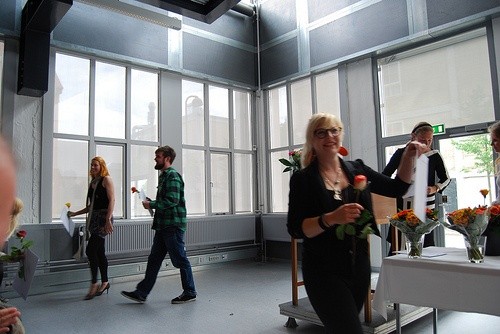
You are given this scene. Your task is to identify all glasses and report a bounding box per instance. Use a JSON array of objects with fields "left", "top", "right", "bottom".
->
[{"left": 313, "top": 127, "right": 341, "bottom": 139}]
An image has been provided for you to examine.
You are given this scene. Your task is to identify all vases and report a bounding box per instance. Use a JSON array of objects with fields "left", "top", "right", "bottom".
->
[
  {"left": 404, "top": 234, "right": 424, "bottom": 259},
  {"left": 464, "top": 236, "right": 486, "bottom": 263}
]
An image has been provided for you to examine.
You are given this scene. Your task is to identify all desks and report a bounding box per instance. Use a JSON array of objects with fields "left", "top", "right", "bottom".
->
[
  {"left": 292, "top": 193, "right": 398, "bottom": 322},
  {"left": 373, "top": 246, "right": 500, "bottom": 334}
]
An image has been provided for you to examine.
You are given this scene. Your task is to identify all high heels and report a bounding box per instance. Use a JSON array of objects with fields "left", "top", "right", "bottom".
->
[
  {"left": 84, "top": 283, "right": 100, "bottom": 300},
  {"left": 97, "top": 282, "right": 110, "bottom": 296}
]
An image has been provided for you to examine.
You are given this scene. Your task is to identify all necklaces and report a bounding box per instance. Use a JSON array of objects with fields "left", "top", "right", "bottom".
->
[{"left": 320, "top": 167, "right": 342, "bottom": 200}]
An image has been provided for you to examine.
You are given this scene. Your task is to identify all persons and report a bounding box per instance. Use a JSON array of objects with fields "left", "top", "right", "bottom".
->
[
  {"left": 120, "top": 145, "right": 196, "bottom": 304},
  {"left": 488, "top": 121, "right": 500, "bottom": 206},
  {"left": 68, "top": 156, "right": 116, "bottom": 301},
  {"left": 381, "top": 122, "right": 450, "bottom": 255},
  {"left": 287, "top": 113, "right": 428, "bottom": 334},
  {"left": 0, "top": 137, "right": 21, "bottom": 334}
]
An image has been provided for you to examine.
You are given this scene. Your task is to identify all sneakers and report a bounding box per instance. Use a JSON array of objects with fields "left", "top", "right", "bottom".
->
[
  {"left": 121, "top": 290, "right": 145, "bottom": 304},
  {"left": 171, "top": 291, "right": 196, "bottom": 303}
]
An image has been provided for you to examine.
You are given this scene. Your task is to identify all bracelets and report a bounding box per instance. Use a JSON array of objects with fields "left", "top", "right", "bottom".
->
[{"left": 319, "top": 214, "right": 329, "bottom": 230}]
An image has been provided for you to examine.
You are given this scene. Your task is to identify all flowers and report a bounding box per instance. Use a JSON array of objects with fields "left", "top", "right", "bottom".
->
[
  {"left": 446, "top": 205, "right": 500, "bottom": 260},
  {"left": 480, "top": 189, "right": 489, "bottom": 204},
  {"left": 279, "top": 149, "right": 302, "bottom": 173},
  {"left": 132, "top": 187, "right": 139, "bottom": 194},
  {"left": 336, "top": 175, "right": 374, "bottom": 265},
  {"left": 392, "top": 208, "right": 439, "bottom": 256},
  {"left": 0, "top": 230, "right": 33, "bottom": 281},
  {"left": 66, "top": 202, "right": 71, "bottom": 228}
]
